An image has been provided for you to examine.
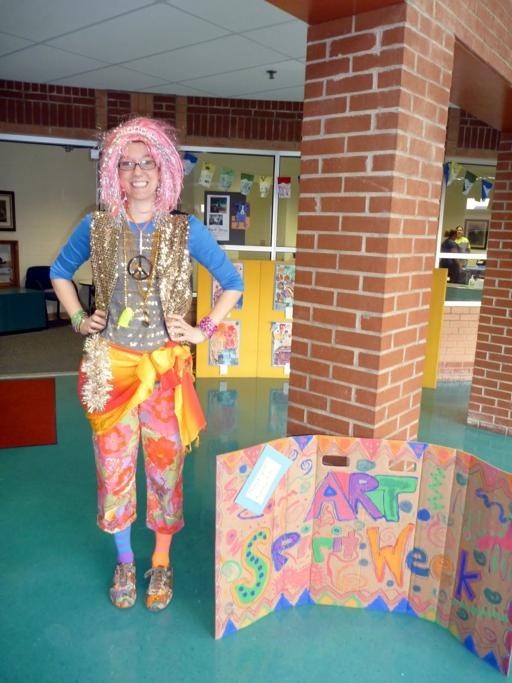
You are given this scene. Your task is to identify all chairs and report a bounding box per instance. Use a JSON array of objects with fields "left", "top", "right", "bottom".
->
[{"left": 25, "top": 266, "right": 77, "bottom": 324}]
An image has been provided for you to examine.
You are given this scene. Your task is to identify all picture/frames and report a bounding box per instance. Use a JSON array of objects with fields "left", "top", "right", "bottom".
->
[
  {"left": 0, "top": 189, "right": 16, "bottom": 232},
  {"left": 464, "top": 218, "right": 489, "bottom": 250},
  {"left": 0, "top": 240, "right": 20, "bottom": 288}
]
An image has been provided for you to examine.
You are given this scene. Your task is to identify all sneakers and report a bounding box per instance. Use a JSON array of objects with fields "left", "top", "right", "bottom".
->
[
  {"left": 143, "top": 561, "right": 174, "bottom": 611},
  {"left": 109, "top": 559, "right": 137, "bottom": 608}
]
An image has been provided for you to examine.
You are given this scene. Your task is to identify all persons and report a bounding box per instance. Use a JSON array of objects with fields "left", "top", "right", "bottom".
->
[
  {"left": 49, "top": 117, "right": 244, "bottom": 610},
  {"left": 442, "top": 229, "right": 463, "bottom": 283},
  {"left": 455, "top": 225, "right": 471, "bottom": 283}
]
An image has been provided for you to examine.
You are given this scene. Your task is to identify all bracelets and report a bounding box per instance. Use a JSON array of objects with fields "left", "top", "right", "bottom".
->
[
  {"left": 199, "top": 316, "right": 218, "bottom": 338},
  {"left": 71, "top": 313, "right": 87, "bottom": 331}
]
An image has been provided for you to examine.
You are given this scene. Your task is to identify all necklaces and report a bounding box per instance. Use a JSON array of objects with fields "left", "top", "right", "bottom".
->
[{"left": 119, "top": 199, "right": 161, "bottom": 327}]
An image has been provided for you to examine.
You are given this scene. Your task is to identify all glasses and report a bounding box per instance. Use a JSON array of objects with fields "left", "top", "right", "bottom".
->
[{"left": 117, "top": 157, "right": 157, "bottom": 172}]
